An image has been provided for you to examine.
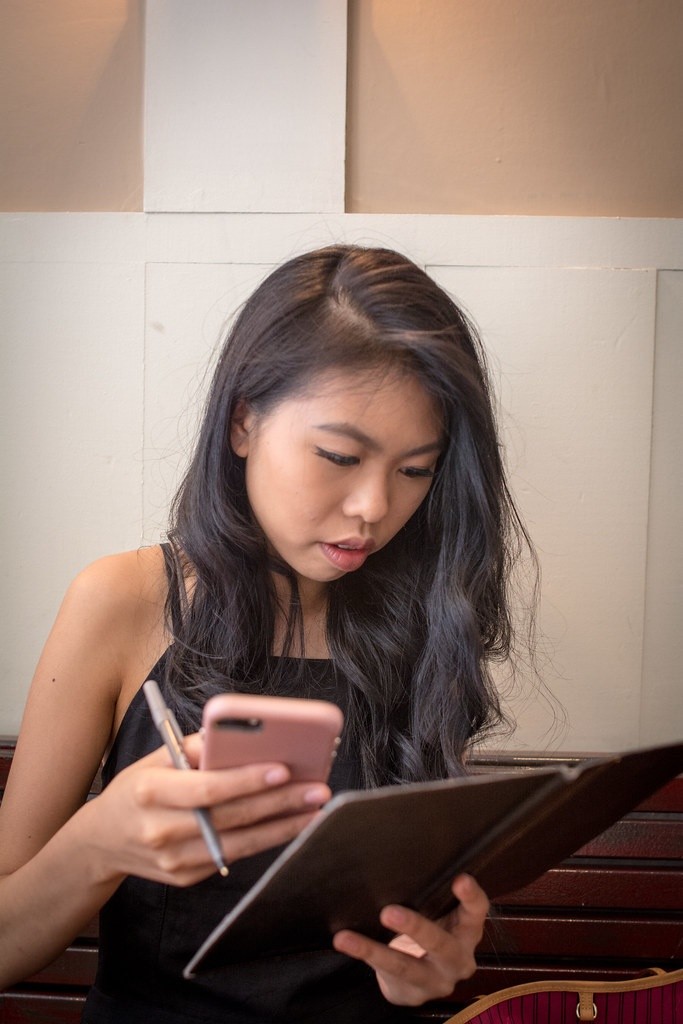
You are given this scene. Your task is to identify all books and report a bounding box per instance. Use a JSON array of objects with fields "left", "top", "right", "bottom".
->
[{"left": 182, "top": 742, "right": 683, "bottom": 984}]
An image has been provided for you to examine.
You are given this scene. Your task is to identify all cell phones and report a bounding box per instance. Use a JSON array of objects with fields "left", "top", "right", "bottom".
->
[{"left": 200, "top": 695, "right": 343, "bottom": 819}]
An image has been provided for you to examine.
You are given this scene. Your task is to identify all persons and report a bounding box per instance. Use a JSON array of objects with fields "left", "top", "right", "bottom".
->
[{"left": 1, "top": 241, "right": 562, "bottom": 1024}]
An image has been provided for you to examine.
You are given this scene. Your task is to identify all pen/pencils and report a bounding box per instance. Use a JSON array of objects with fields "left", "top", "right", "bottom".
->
[{"left": 143, "top": 680, "right": 230, "bottom": 879}]
